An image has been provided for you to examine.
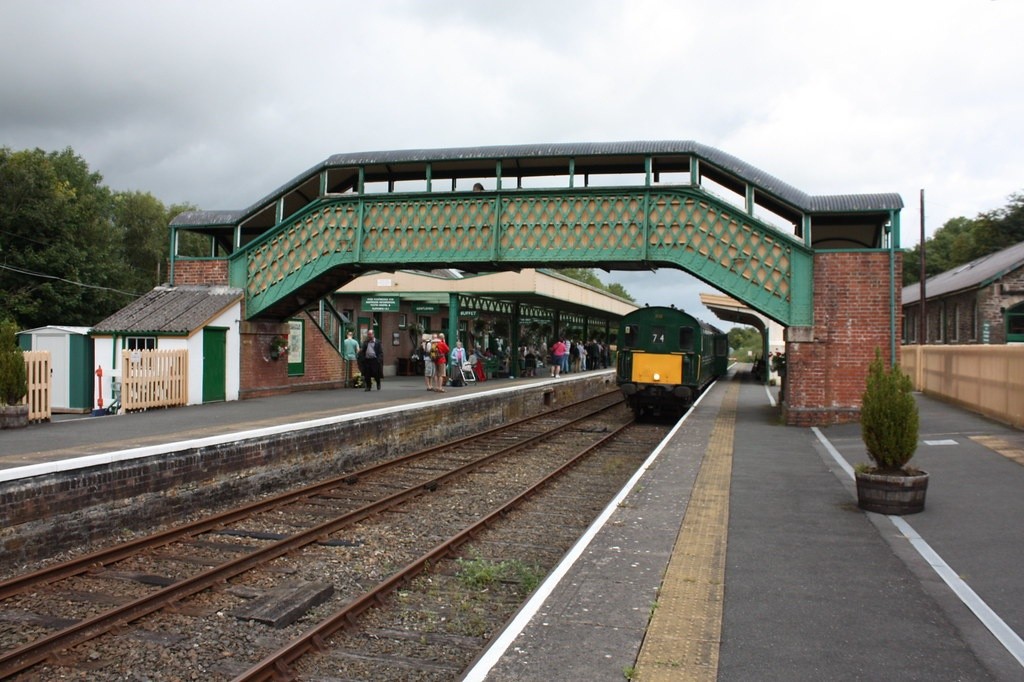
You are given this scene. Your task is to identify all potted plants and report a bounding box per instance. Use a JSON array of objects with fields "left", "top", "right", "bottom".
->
[
  {"left": 1, "top": 317, "right": 31, "bottom": 428},
  {"left": 397, "top": 322, "right": 425, "bottom": 376},
  {"left": 855, "top": 350, "right": 931, "bottom": 518}
]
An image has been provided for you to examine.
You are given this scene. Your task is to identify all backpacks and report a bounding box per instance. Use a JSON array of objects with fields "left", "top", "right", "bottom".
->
[{"left": 429, "top": 343, "right": 444, "bottom": 362}]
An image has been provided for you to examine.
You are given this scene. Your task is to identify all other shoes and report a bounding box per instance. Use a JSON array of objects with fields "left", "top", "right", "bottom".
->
[
  {"left": 551, "top": 373, "right": 561, "bottom": 377},
  {"left": 377, "top": 382, "right": 381, "bottom": 390},
  {"left": 434, "top": 388, "right": 447, "bottom": 393},
  {"left": 364, "top": 388, "right": 371, "bottom": 391},
  {"left": 427, "top": 386, "right": 433, "bottom": 391}
]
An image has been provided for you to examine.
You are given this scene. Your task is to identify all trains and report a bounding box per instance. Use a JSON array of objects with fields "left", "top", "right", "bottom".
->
[{"left": 616, "top": 302, "right": 731, "bottom": 420}]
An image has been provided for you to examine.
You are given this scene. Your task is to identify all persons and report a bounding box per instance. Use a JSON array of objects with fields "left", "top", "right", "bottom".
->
[
  {"left": 473, "top": 183, "right": 483, "bottom": 192},
  {"left": 343, "top": 331, "right": 359, "bottom": 360},
  {"left": 360, "top": 329, "right": 385, "bottom": 391},
  {"left": 421, "top": 330, "right": 606, "bottom": 393}
]
